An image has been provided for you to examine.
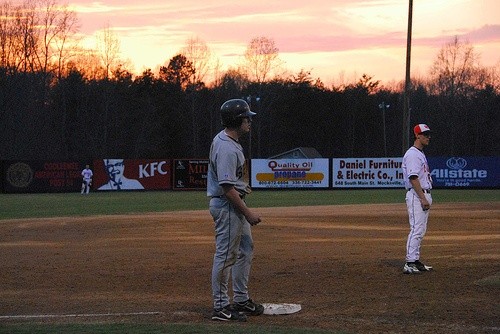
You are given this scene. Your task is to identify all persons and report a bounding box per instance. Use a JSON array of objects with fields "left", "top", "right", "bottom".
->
[
  {"left": 81, "top": 164, "right": 93, "bottom": 194},
  {"left": 206, "top": 99, "right": 264, "bottom": 321},
  {"left": 401, "top": 124, "right": 433, "bottom": 274}
]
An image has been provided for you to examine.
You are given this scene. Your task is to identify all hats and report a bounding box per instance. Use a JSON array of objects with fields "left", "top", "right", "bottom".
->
[{"left": 413, "top": 123, "right": 432, "bottom": 135}]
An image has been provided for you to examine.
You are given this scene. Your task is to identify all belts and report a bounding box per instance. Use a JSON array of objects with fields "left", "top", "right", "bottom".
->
[
  {"left": 211, "top": 195, "right": 246, "bottom": 199},
  {"left": 409, "top": 188, "right": 430, "bottom": 194}
]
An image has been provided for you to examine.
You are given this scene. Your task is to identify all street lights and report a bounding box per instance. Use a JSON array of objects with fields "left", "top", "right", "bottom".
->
[
  {"left": 241, "top": 95, "right": 261, "bottom": 160},
  {"left": 379, "top": 101, "right": 390, "bottom": 157}
]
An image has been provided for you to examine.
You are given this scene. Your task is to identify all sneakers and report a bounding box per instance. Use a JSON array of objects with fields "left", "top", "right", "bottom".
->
[
  {"left": 233, "top": 299, "right": 264, "bottom": 316},
  {"left": 212, "top": 305, "right": 248, "bottom": 322},
  {"left": 415, "top": 260, "right": 433, "bottom": 271},
  {"left": 402, "top": 263, "right": 425, "bottom": 274}
]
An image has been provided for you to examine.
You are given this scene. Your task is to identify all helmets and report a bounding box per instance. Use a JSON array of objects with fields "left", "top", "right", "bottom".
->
[{"left": 221, "top": 99, "right": 256, "bottom": 126}]
